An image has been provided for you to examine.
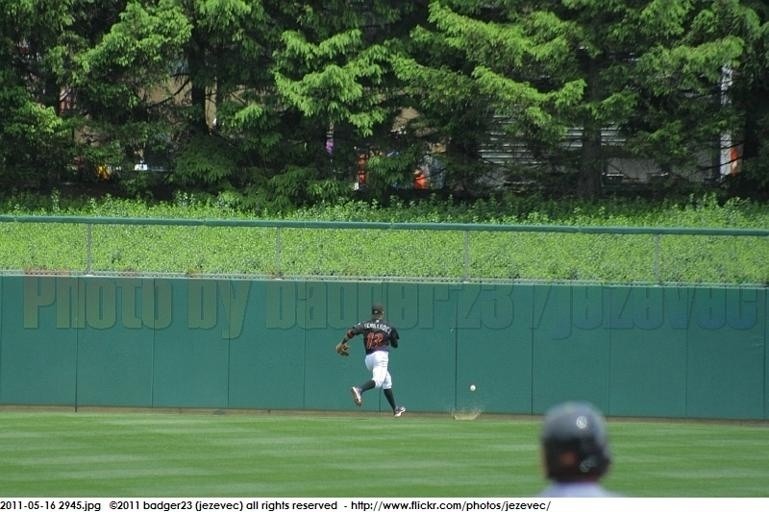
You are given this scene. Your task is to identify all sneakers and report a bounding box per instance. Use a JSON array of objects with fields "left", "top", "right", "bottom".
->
[
  {"left": 393, "top": 406, "right": 406, "bottom": 417},
  {"left": 351, "top": 386, "right": 363, "bottom": 406}
]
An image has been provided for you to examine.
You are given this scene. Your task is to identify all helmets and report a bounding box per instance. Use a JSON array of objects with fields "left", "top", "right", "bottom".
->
[{"left": 539, "top": 401, "right": 611, "bottom": 483}]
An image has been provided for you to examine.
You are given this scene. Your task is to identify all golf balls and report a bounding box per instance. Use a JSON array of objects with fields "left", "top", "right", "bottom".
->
[{"left": 470, "top": 385, "right": 476, "bottom": 391}]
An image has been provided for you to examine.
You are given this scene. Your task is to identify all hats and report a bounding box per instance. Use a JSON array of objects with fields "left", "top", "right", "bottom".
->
[{"left": 371, "top": 304, "right": 384, "bottom": 315}]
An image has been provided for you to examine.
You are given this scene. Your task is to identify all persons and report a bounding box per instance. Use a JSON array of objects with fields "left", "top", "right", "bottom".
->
[
  {"left": 533, "top": 400, "right": 624, "bottom": 498},
  {"left": 336, "top": 304, "right": 407, "bottom": 417}
]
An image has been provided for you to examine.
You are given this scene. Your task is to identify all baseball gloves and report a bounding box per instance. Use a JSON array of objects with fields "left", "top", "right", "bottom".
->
[{"left": 336, "top": 343, "right": 350, "bottom": 355}]
